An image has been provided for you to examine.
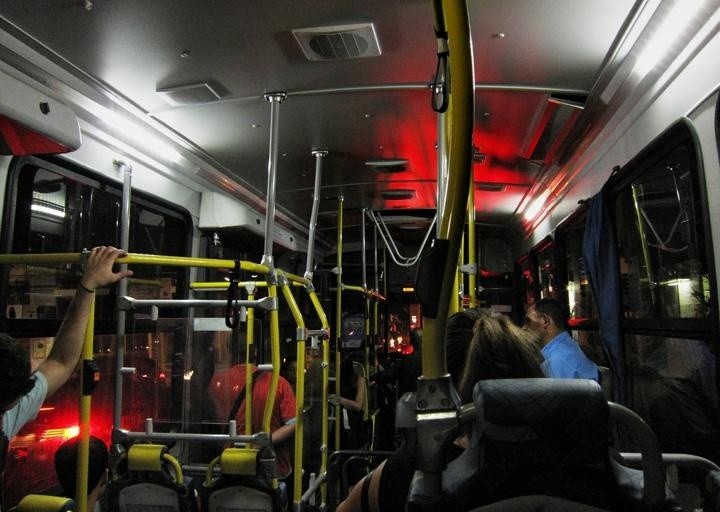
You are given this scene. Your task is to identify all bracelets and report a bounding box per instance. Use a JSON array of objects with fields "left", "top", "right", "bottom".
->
[{"left": 76, "top": 281, "right": 96, "bottom": 294}]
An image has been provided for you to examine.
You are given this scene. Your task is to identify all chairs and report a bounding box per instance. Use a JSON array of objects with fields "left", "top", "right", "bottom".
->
[{"left": 2, "top": 346, "right": 666, "bottom": 511}]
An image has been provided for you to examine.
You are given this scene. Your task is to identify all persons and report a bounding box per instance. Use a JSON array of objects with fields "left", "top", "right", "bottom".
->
[
  {"left": 278, "top": 322, "right": 429, "bottom": 480},
  {"left": 203, "top": 332, "right": 296, "bottom": 511},
  {"left": 49, "top": 434, "right": 113, "bottom": 512},
  {"left": 0, "top": 241, "right": 133, "bottom": 512},
  {"left": 332, "top": 306, "right": 549, "bottom": 511},
  {"left": 517, "top": 295, "right": 600, "bottom": 382}
]
